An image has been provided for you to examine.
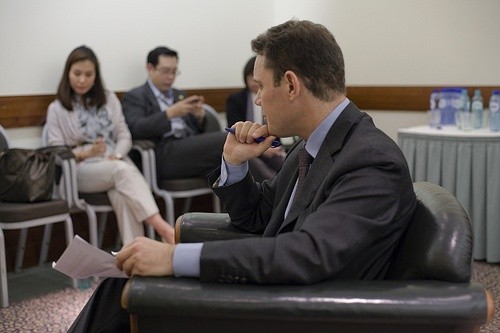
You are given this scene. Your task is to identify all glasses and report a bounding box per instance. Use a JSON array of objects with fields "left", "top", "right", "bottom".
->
[{"left": 152, "top": 65, "right": 180, "bottom": 76}]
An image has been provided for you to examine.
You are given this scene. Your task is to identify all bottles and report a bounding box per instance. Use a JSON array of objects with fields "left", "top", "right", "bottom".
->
[{"left": 427, "top": 89, "right": 500, "bottom": 132}]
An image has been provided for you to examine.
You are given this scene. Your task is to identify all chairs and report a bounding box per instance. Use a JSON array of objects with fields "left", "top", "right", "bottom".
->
[{"left": 0, "top": 103, "right": 228, "bottom": 309}]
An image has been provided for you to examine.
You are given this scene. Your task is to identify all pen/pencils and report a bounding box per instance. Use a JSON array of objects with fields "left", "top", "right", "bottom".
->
[{"left": 224, "top": 127, "right": 282, "bottom": 148}]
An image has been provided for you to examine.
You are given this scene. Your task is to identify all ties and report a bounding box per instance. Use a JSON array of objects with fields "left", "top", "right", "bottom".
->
[{"left": 296, "top": 146, "right": 314, "bottom": 192}]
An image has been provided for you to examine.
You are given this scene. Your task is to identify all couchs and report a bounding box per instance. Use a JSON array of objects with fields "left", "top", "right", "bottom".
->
[{"left": 119, "top": 182, "right": 494, "bottom": 333}]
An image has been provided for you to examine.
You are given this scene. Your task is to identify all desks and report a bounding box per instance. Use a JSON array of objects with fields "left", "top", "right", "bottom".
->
[{"left": 396, "top": 123, "right": 500, "bottom": 264}]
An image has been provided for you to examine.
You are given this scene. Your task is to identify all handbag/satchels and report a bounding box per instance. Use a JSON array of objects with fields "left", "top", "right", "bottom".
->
[{"left": 0, "top": 149, "right": 59, "bottom": 204}]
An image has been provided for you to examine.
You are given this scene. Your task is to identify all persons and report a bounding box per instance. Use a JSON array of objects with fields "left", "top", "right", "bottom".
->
[
  {"left": 67, "top": 19, "right": 418, "bottom": 333},
  {"left": 47, "top": 47, "right": 175, "bottom": 246},
  {"left": 226, "top": 56, "right": 265, "bottom": 128},
  {"left": 121, "top": 47, "right": 276, "bottom": 183}
]
